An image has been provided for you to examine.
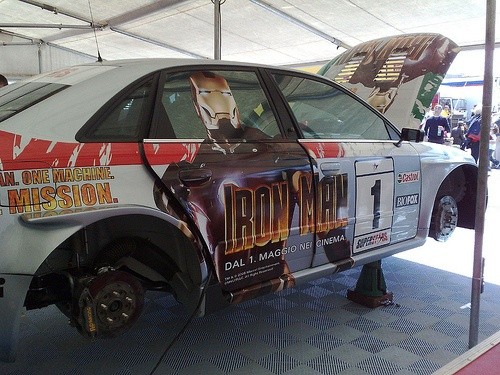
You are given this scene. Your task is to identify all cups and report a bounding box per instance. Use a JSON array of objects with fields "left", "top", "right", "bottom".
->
[
  {"left": 438, "top": 126, "right": 442, "bottom": 136},
  {"left": 447, "top": 133, "right": 451, "bottom": 138}
]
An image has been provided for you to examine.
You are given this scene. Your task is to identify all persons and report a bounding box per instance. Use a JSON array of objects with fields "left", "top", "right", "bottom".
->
[
  {"left": 424, "top": 104, "right": 450, "bottom": 145},
  {"left": 451, "top": 121, "right": 466, "bottom": 151},
  {"left": 464, "top": 104, "right": 482, "bottom": 164},
  {"left": 420, "top": 102, "right": 500, "bottom": 177}
]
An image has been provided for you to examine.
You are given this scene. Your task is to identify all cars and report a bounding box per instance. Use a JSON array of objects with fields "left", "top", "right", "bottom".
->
[
  {"left": 439, "top": 96, "right": 500, "bottom": 123},
  {"left": 0, "top": 32, "right": 488, "bottom": 363}
]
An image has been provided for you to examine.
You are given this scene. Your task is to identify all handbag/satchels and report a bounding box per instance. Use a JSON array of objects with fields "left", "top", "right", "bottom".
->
[{"left": 465, "top": 131, "right": 480, "bottom": 142}]
